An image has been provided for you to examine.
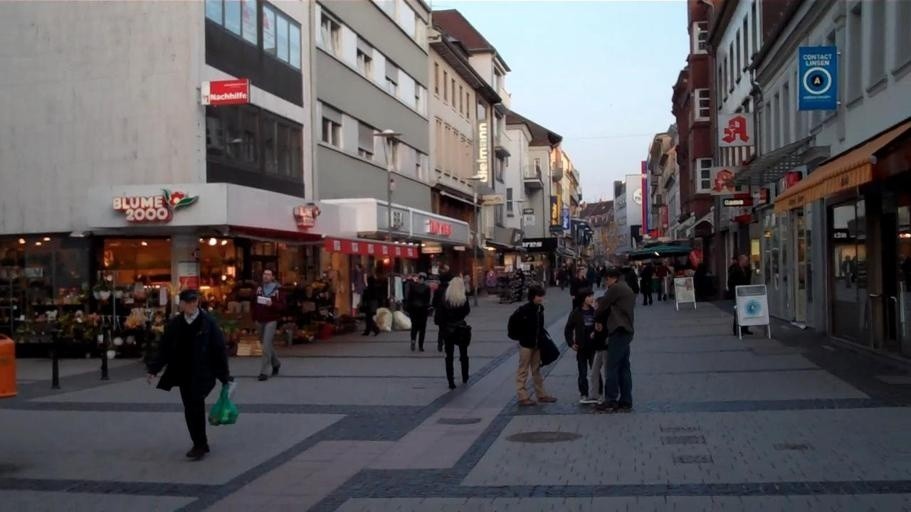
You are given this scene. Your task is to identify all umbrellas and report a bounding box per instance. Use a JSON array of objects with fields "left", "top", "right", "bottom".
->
[{"left": 629, "top": 244, "right": 694, "bottom": 276}]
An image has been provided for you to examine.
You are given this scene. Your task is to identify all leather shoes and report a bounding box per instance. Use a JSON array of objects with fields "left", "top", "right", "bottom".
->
[
  {"left": 273, "top": 364, "right": 280, "bottom": 375},
  {"left": 743, "top": 328, "right": 754, "bottom": 334},
  {"left": 540, "top": 396, "right": 556, "bottom": 402},
  {"left": 186, "top": 444, "right": 209, "bottom": 458},
  {"left": 259, "top": 373, "right": 268, "bottom": 381},
  {"left": 519, "top": 399, "right": 537, "bottom": 406}
]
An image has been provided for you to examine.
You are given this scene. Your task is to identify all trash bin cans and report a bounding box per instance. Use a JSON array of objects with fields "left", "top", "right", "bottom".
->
[{"left": 651, "top": 277, "right": 660, "bottom": 292}]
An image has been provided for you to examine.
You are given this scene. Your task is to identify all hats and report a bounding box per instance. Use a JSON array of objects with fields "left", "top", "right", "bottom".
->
[{"left": 179, "top": 289, "right": 200, "bottom": 302}]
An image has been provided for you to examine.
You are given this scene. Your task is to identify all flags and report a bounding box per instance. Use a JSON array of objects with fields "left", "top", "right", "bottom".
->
[
  {"left": 710, "top": 165, "right": 738, "bottom": 195},
  {"left": 718, "top": 110, "right": 753, "bottom": 149},
  {"left": 795, "top": 44, "right": 838, "bottom": 112}
]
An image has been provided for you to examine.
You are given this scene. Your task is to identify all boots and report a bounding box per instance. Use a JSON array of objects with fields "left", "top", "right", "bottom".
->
[
  {"left": 446, "top": 356, "right": 457, "bottom": 390},
  {"left": 460, "top": 357, "right": 469, "bottom": 383}
]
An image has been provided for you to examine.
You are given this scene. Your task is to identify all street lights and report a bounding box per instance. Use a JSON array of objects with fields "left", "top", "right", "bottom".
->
[
  {"left": 372, "top": 127, "right": 404, "bottom": 333},
  {"left": 471, "top": 195, "right": 529, "bottom": 305}
]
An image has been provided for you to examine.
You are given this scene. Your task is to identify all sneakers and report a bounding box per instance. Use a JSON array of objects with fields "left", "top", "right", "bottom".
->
[{"left": 579, "top": 393, "right": 630, "bottom": 411}]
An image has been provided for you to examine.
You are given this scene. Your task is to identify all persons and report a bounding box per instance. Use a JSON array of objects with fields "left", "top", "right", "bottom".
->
[
  {"left": 852, "top": 255, "right": 860, "bottom": 282},
  {"left": 144, "top": 288, "right": 237, "bottom": 461},
  {"left": 728, "top": 254, "right": 756, "bottom": 337},
  {"left": 580, "top": 297, "right": 610, "bottom": 405},
  {"left": 619, "top": 266, "right": 642, "bottom": 296},
  {"left": 564, "top": 287, "right": 604, "bottom": 406},
  {"left": 357, "top": 258, "right": 472, "bottom": 389},
  {"left": 594, "top": 268, "right": 636, "bottom": 410},
  {"left": 515, "top": 285, "right": 559, "bottom": 407},
  {"left": 517, "top": 255, "right": 713, "bottom": 307},
  {"left": 251, "top": 267, "right": 287, "bottom": 383},
  {"left": 842, "top": 252, "right": 856, "bottom": 289}
]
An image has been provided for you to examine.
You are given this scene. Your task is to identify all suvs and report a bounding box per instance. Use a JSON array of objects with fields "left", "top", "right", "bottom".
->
[{"left": 848, "top": 254, "right": 867, "bottom": 290}]
